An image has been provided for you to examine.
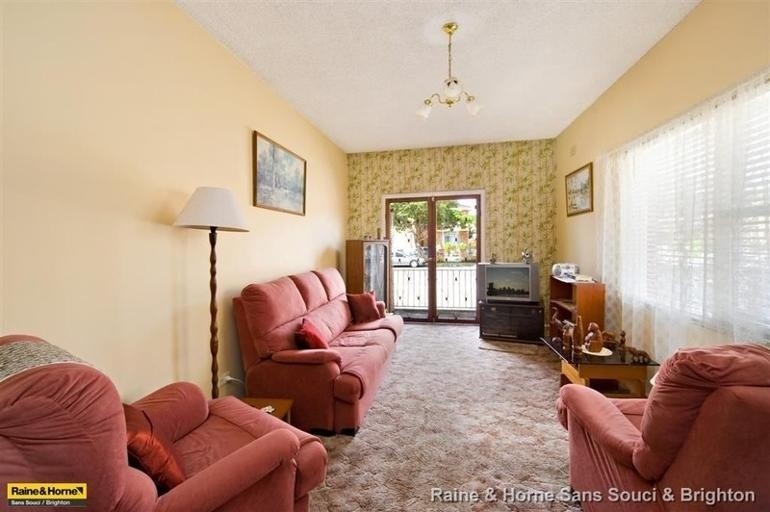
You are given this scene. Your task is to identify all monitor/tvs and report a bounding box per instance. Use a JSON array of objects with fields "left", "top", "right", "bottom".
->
[{"left": 476, "top": 262, "right": 538, "bottom": 302}]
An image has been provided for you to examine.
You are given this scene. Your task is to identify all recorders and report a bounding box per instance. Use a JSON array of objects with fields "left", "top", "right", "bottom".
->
[{"left": 552, "top": 263, "right": 581, "bottom": 277}]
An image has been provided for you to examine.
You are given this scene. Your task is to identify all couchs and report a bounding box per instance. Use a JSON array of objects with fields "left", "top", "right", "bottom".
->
[{"left": 233, "top": 268, "right": 403, "bottom": 436}]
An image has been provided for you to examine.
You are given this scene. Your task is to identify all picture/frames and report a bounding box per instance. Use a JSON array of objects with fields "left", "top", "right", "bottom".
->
[
  {"left": 565, "top": 162, "right": 594, "bottom": 217},
  {"left": 253, "top": 131, "right": 307, "bottom": 216}
]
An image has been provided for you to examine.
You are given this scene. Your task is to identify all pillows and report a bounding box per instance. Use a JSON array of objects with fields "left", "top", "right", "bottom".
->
[
  {"left": 122, "top": 403, "right": 184, "bottom": 490},
  {"left": 297, "top": 318, "right": 328, "bottom": 349},
  {"left": 346, "top": 290, "right": 380, "bottom": 323}
]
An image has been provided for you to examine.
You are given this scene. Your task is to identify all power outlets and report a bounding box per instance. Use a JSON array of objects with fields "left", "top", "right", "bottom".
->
[{"left": 220, "top": 371, "right": 232, "bottom": 388}]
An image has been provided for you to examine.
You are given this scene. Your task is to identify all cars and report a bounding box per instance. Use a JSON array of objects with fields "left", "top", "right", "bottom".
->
[
  {"left": 391, "top": 245, "right": 430, "bottom": 268},
  {"left": 444, "top": 252, "right": 462, "bottom": 263}
]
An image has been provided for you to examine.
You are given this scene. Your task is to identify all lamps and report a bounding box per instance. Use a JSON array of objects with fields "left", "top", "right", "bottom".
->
[
  {"left": 176, "top": 188, "right": 250, "bottom": 399},
  {"left": 417, "top": 23, "right": 481, "bottom": 118}
]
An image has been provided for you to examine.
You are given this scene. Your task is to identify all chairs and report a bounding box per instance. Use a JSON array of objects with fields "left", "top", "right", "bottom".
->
[
  {"left": 556, "top": 343, "right": 770, "bottom": 512},
  {"left": 0, "top": 335, "right": 327, "bottom": 512}
]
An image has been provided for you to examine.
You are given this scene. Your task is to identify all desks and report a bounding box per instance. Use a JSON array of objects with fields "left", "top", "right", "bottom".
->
[
  {"left": 241, "top": 398, "right": 295, "bottom": 425},
  {"left": 540, "top": 335, "right": 660, "bottom": 397}
]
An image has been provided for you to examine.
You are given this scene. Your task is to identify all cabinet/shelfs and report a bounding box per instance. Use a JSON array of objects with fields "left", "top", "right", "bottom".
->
[
  {"left": 478, "top": 300, "right": 544, "bottom": 345},
  {"left": 549, "top": 275, "right": 605, "bottom": 342},
  {"left": 346, "top": 239, "right": 390, "bottom": 312}
]
